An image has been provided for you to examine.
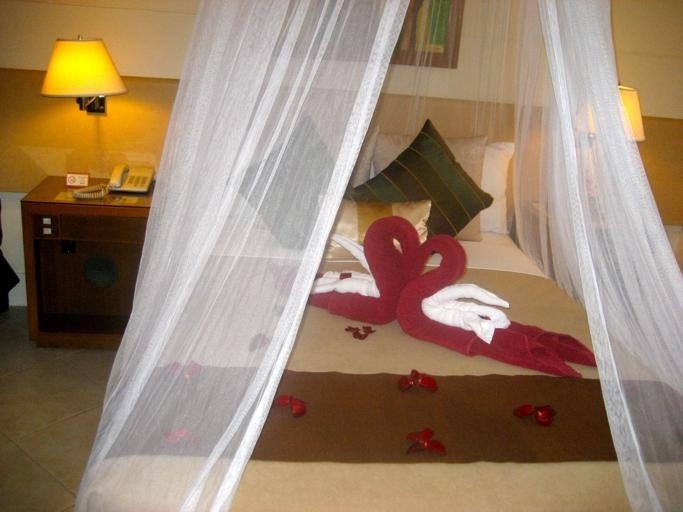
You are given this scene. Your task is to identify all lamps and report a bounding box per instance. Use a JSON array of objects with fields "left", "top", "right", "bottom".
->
[
  {"left": 582, "top": 85, "right": 646, "bottom": 162},
  {"left": 39, "top": 34, "right": 130, "bottom": 117}
]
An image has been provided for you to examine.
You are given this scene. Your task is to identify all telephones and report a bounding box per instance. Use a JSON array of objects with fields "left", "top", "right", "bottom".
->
[{"left": 73, "top": 164, "right": 154, "bottom": 200}]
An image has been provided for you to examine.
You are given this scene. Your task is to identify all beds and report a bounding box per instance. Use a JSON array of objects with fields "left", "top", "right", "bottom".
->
[{"left": 75, "top": 233, "right": 680, "bottom": 511}]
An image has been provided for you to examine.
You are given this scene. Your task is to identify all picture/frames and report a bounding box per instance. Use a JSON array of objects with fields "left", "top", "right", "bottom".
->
[{"left": 273, "top": 1, "right": 465, "bottom": 70}]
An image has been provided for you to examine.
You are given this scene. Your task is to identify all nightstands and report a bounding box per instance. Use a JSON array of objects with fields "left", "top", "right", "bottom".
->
[{"left": 20, "top": 176, "right": 157, "bottom": 350}]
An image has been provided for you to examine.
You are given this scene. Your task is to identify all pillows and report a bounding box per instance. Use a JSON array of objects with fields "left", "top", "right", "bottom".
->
[
  {"left": 479, "top": 141, "right": 516, "bottom": 241},
  {"left": 346, "top": 125, "right": 379, "bottom": 189},
  {"left": 369, "top": 135, "right": 489, "bottom": 243},
  {"left": 228, "top": 116, "right": 357, "bottom": 250},
  {"left": 350, "top": 119, "right": 497, "bottom": 247},
  {"left": 313, "top": 195, "right": 431, "bottom": 278}
]
